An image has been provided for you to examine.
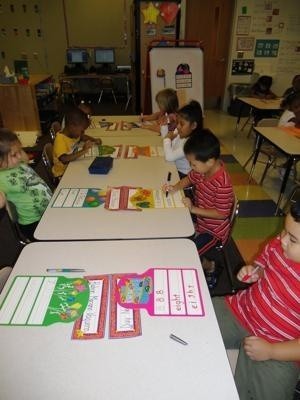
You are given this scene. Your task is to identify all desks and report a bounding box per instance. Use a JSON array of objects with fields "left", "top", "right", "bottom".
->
[
  {"left": 0, "top": 238, "right": 241, "bottom": 399},
  {"left": 58, "top": 70, "right": 132, "bottom": 113},
  {"left": 30, "top": 136, "right": 193, "bottom": 240},
  {"left": 82, "top": 114, "right": 163, "bottom": 136}
]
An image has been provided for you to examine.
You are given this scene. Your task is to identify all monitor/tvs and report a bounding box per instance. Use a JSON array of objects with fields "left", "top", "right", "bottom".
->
[
  {"left": 66, "top": 48, "right": 88, "bottom": 64},
  {"left": 94, "top": 48, "right": 115, "bottom": 64}
]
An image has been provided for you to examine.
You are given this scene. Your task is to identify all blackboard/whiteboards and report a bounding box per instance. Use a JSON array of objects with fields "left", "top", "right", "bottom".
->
[{"left": 147, "top": 45, "right": 204, "bottom": 114}]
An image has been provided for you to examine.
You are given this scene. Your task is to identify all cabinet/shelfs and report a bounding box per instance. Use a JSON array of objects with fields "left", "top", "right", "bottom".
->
[{"left": 0, "top": 73, "right": 55, "bottom": 132}]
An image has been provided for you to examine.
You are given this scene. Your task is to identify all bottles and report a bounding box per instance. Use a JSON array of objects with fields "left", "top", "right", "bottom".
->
[{"left": 14, "top": 67, "right": 29, "bottom": 85}]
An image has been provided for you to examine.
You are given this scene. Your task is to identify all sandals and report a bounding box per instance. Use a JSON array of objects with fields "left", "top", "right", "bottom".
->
[{"left": 205, "top": 259, "right": 217, "bottom": 289}]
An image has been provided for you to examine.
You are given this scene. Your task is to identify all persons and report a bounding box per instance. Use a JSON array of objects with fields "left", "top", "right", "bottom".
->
[
  {"left": 249, "top": 76, "right": 276, "bottom": 141},
  {"left": 51, "top": 107, "right": 102, "bottom": 189},
  {"left": 138, "top": 89, "right": 180, "bottom": 139},
  {"left": 0, "top": 129, "right": 53, "bottom": 241},
  {"left": 161, "top": 127, "right": 236, "bottom": 290},
  {"left": 158, "top": 99, "right": 204, "bottom": 223},
  {"left": 283, "top": 75, "right": 300, "bottom": 98},
  {"left": 211, "top": 200, "right": 300, "bottom": 400},
  {"left": 77, "top": 103, "right": 93, "bottom": 117},
  {"left": 277, "top": 92, "right": 300, "bottom": 182}
]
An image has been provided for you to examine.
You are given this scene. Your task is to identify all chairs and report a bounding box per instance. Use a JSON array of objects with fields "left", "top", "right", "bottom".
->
[
  {"left": 38, "top": 141, "right": 61, "bottom": 188},
  {"left": 59, "top": 77, "right": 82, "bottom": 107},
  {"left": 122, "top": 75, "right": 133, "bottom": 112},
  {"left": 3, "top": 199, "right": 32, "bottom": 247},
  {"left": 236, "top": 89, "right": 300, "bottom": 216},
  {"left": 96, "top": 74, "right": 119, "bottom": 105},
  {"left": 208, "top": 194, "right": 242, "bottom": 290},
  {"left": 49, "top": 120, "right": 62, "bottom": 142}
]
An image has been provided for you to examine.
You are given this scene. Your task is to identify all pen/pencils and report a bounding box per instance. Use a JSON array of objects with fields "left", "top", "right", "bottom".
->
[
  {"left": 241, "top": 265, "right": 260, "bottom": 282},
  {"left": 47, "top": 268, "right": 86, "bottom": 272},
  {"left": 141, "top": 113, "right": 143, "bottom": 124},
  {"left": 166, "top": 172, "right": 171, "bottom": 198}
]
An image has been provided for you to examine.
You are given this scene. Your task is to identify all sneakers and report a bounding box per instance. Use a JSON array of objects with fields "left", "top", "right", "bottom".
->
[{"left": 280, "top": 164, "right": 298, "bottom": 180}]
